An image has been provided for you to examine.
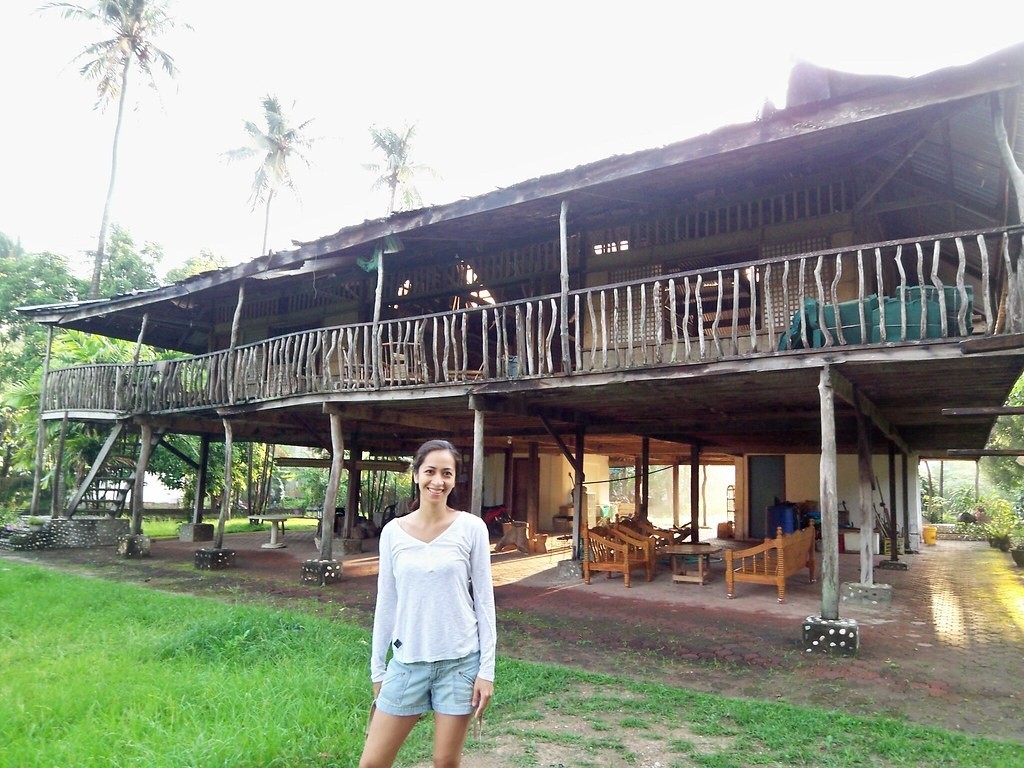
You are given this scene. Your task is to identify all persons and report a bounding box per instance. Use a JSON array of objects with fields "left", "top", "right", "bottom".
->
[{"left": 357, "top": 438, "right": 498, "bottom": 768}]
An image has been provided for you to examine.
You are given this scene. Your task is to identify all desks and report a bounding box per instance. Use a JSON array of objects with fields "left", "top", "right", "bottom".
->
[
  {"left": 247, "top": 513, "right": 287, "bottom": 550},
  {"left": 660, "top": 544, "right": 724, "bottom": 587}
]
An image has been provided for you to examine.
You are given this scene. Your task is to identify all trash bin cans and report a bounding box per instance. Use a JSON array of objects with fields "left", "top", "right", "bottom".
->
[
  {"left": 923, "top": 527, "right": 937, "bottom": 546},
  {"left": 334, "top": 508, "right": 345, "bottom": 533}
]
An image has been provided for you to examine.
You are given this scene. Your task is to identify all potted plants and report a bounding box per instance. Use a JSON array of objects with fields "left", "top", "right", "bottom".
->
[{"left": 27, "top": 515, "right": 46, "bottom": 533}]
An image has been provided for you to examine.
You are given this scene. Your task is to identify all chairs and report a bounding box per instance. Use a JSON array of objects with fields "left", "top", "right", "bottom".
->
[
  {"left": 613, "top": 512, "right": 675, "bottom": 578},
  {"left": 582, "top": 517, "right": 654, "bottom": 590}
]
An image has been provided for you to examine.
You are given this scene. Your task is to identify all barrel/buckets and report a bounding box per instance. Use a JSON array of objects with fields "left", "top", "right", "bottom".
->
[
  {"left": 923, "top": 526, "right": 938, "bottom": 545},
  {"left": 502, "top": 354, "right": 520, "bottom": 378},
  {"left": 764, "top": 505, "right": 794, "bottom": 550}
]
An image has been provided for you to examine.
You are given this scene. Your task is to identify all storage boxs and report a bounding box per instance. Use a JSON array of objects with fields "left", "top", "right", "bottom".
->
[
  {"left": 884, "top": 537, "right": 905, "bottom": 556},
  {"left": 843, "top": 531, "right": 881, "bottom": 554}
]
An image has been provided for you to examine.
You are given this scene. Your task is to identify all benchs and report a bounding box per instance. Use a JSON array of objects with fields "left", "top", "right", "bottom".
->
[{"left": 727, "top": 518, "right": 818, "bottom": 603}]
